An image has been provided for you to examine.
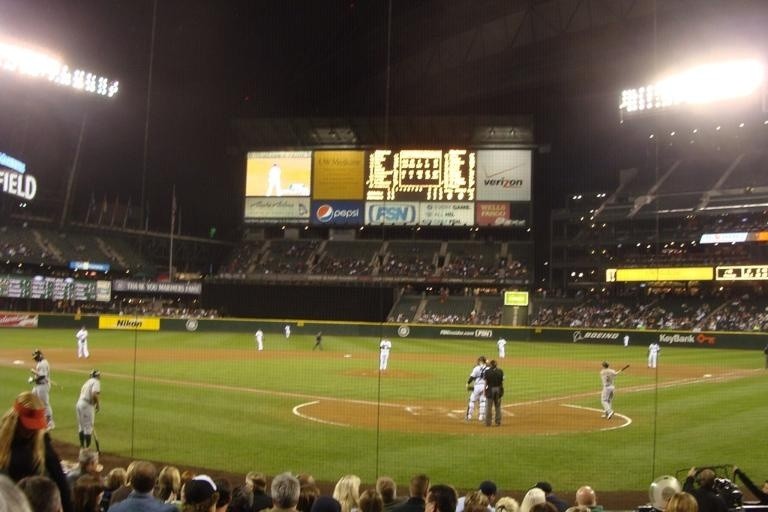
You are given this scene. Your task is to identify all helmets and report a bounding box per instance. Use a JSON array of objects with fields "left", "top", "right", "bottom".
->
[{"left": 89, "top": 369, "right": 102, "bottom": 378}]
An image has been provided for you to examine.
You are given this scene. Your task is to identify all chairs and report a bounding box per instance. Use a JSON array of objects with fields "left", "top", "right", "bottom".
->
[
  {"left": 588, "top": 155, "right": 768, "bottom": 267},
  {"left": 386, "top": 292, "right": 767, "bottom": 334},
  {"left": 0, "top": 224, "right": 154, "bottom": 276},
  {"left": 215, "top": 238, "right": 538, "bottom": 286}
]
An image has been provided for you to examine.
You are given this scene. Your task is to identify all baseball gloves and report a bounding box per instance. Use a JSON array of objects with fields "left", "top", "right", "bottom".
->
[{"left": 466, "top": 386, "right": 474, "bottom": 390}]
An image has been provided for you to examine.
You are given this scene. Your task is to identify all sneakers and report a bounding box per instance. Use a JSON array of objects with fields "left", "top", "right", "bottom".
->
[
  {"left": 47, "top": 422, "right": 56, "bottom": 433},
  {"left": 601, "top": 410, "right": 615, "bottom": 421}
]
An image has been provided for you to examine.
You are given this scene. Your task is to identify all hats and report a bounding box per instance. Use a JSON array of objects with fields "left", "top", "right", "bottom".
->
[
  {"left": 180, "top": 472, "right": 219, "bottom": 508},
  {"left": 31, "top": 348, "right": 42, "bottom": 360}
]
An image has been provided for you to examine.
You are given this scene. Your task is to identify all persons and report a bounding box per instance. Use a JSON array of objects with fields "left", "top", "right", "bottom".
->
[{"left": 0, "top": 187, "right": 768, "bottom": 512}]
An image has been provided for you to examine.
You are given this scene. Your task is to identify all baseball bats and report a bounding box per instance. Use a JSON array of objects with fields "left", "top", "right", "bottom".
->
[
  {"left": 31, "top": 369, "right": 62, "bottom": 390},
  {"left": 93, "top": 430, "right": 102, "bottom": 456},
  {"left": 618, "top": 364, "right": 629, "bottom": 373}
]
